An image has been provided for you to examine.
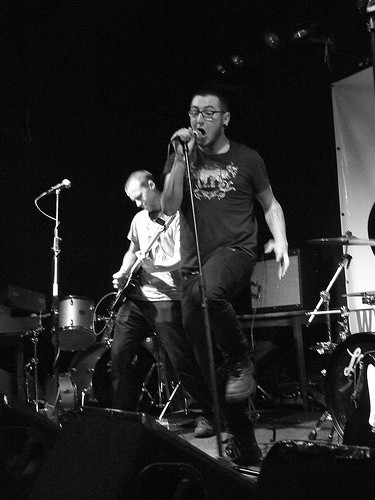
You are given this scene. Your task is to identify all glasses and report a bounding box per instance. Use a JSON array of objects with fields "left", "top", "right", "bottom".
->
[{"left": 189, "top": 109, "right": 226, "bottom": 118}]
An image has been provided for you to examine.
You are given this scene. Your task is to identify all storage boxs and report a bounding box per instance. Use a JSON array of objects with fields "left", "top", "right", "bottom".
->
[{"left": 235, "top": 308, "right": 326, "bottom": 414}]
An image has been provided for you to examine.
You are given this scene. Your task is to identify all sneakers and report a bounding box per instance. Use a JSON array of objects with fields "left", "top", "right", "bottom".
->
[
  {"left": 226, "top": 361, "right": 255, "bottom": 401},
  {"left": 224, "top": 447, "right": 263, "bottom": 464},
  {"left": 194, "top": 416, "right": 217, "bottom": 438}
]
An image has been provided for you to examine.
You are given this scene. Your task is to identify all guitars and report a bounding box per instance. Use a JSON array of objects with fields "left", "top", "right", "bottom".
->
[{"left": 109, "top": 216, "right": 174, "bottom": 312}]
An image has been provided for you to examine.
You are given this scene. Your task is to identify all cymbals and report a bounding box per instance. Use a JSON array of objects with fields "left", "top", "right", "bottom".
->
[
  {"left": 341, "top": 291, "right": 375, "bottom": 297},
  {"left": 304, "top": 235, "right": 375, "bottom": 246}
]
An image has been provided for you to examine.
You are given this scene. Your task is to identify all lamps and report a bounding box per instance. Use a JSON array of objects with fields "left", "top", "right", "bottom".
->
[
  {"left": 214, "top": 57, "right": 235, "bottom": 77},
  {"left": 230, "top": 15, "right": 309, "bottom": 68}
]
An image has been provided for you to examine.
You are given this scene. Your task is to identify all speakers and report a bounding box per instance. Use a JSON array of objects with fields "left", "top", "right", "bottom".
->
[
  {"left": 253, "top": 439, "right": 375, "bottom": 499},
  {"left": 246, "top": 246, "right": 312, "bottom": 313},
  {"left": 28, "top": 405, "right": 258, "bottom": 499},
  {"left": 0, "top": 393, "right": 56, "bottom": 500}
]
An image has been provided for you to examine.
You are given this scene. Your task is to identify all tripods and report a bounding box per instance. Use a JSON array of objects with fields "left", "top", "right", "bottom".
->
[{"left": 135, "top": 333, "right": 186, "bottom": 419}]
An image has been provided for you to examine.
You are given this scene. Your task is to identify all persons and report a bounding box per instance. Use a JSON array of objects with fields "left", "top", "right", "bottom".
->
[
  {"left": 160, "top": 86, "right": 290, "bottom": 465},
  {"left": 109, "top": 170, "right": 223, "bottom": 438}
]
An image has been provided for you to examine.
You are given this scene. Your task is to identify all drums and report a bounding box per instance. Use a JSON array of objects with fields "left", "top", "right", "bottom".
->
[
  {"left": 48, "top": 294, "right": 97, "bottom": 351},
  {"left": 70, "top": 342, "right": 171, "bottom": 419},
  {"left": 44, "top": 372, "right": 78, "bottom": 428},
  {"left": 324, "top": 332, "right": 375, "bottom": 439}
]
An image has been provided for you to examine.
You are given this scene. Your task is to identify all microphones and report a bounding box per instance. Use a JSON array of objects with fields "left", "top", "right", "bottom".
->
[
  {"left": 173, "top": 130, "right": 203, "bottom": 141},
  {"left": 47, "top": 179, "right": 71, "bottom": 194}
]
